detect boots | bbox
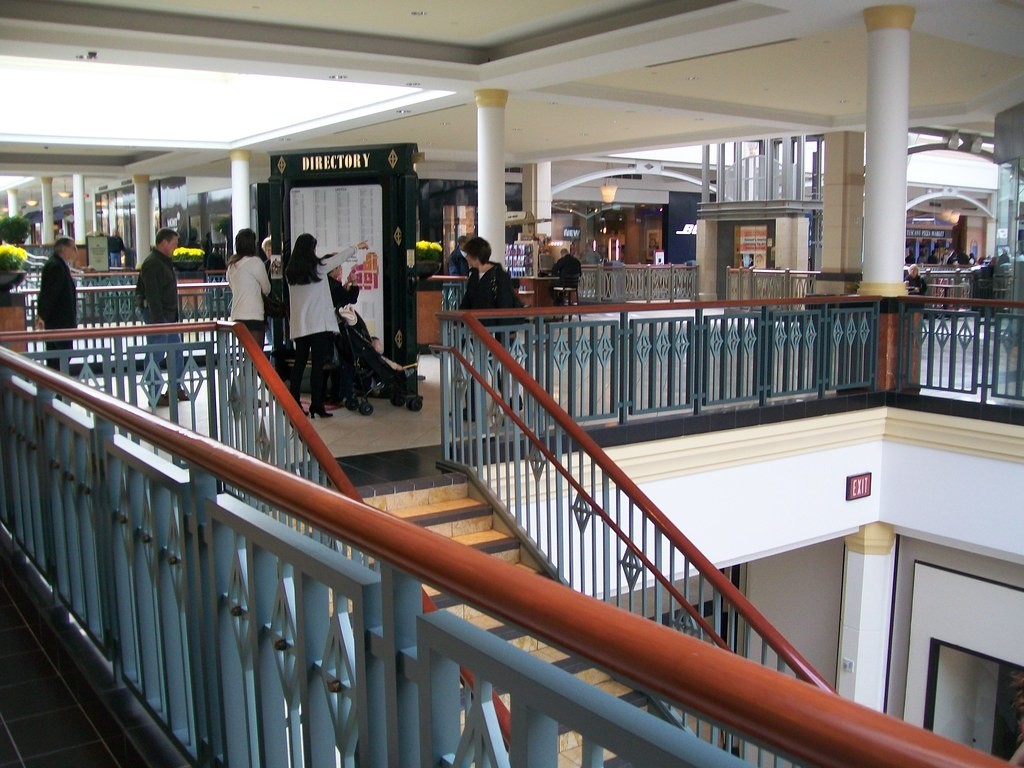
[228,376,269,408]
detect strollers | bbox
[333,305,424,416]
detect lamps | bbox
[599,161,620,204]
[949,131,960,149]
[970,134,983,154]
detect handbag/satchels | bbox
[488,266,524,334]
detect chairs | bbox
[552,273,582,322]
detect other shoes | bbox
[449,408,475,422]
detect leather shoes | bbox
[148,389,189,406]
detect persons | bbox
[108,230,125,267]
[904,264,927,309]
[550,248,582,306]
[34,236,76,403]
[225,228,405,418]
[906,249,976,265]
[447,236,531,422]
[136,226,190,407]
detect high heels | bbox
[283,402,309,416]
[309,404,333,418]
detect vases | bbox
[173,260,202,271]
[0,270,28,294]
[415,259,442,281]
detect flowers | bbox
[0,244,29,271]
[414,240,443,262]
[172,246,205,262]
